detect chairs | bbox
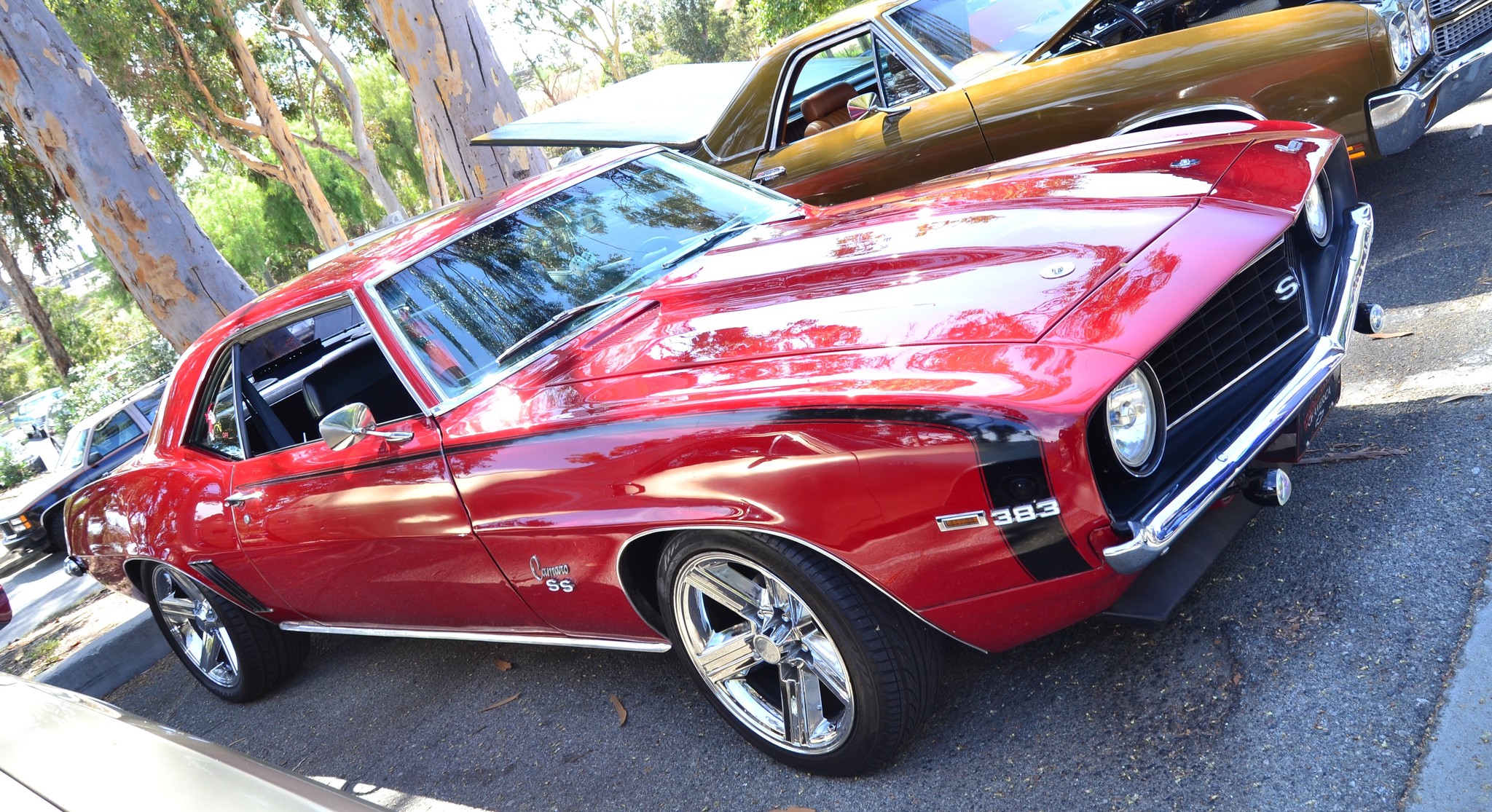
[301,325,457,435]
[801,80,859,137]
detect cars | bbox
[12,385,66,439]
[54,120,1392,782]
[460,0,1449,212]
[0,368,200,556]
